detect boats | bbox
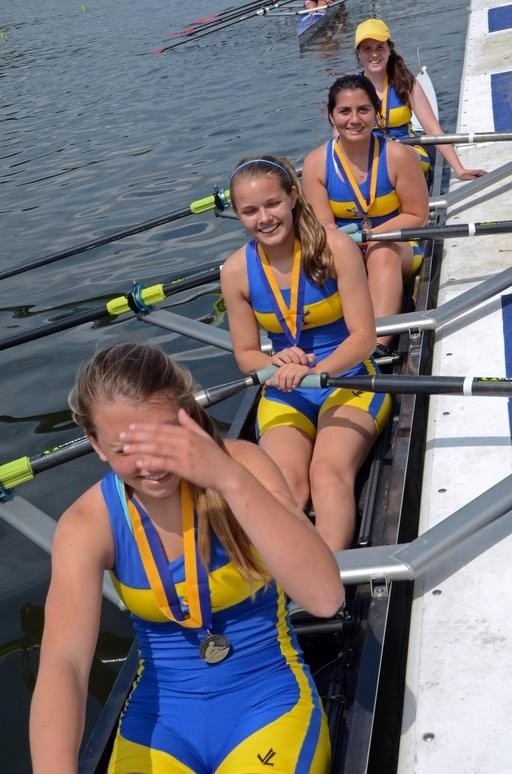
[63,62,453,774]
[294,0,342,47]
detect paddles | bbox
[153,0,292,56]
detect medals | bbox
[359,216,374,231]
[198,634,232,665]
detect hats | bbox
[353,19,392,49]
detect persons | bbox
[221,153,393,559]
[30,343,347,774]
[304,1,331,16]
[351,19,486,184]
[301,73,434,368]
